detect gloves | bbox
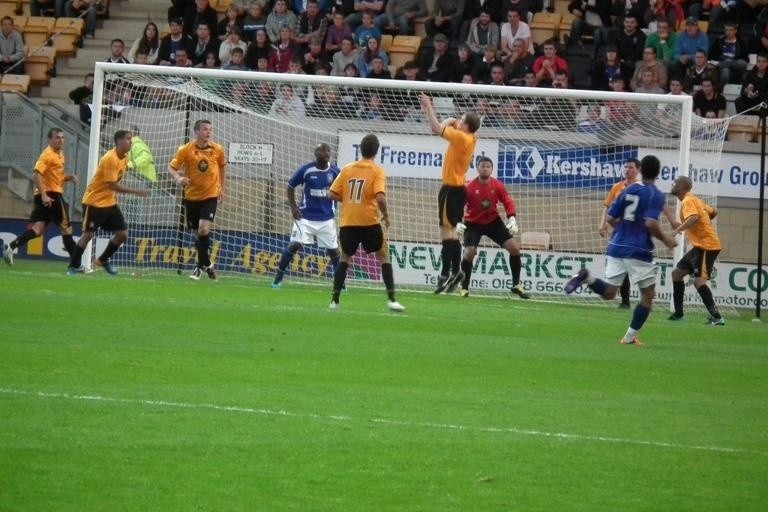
[506,216,519,236]
[456,222,467,236]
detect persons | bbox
[0,16,26,74]
[125,123,158,218]
[418,90,483,295]
[66,130,150,276]
[599,156,682,311]
[15,1,109,49]
[460,158,530,299]
[564,155,678,346]
[270,140,350,288]
[326,135,409,311]
[668,176,726,326]
[3,127,93,272]
[158,0,768,142]
[168,119,227,280]
[69,22,162,129]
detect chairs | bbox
[0,0,767,141]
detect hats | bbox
[686,16,697,24]
[434,33,448,43]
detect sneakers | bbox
[272,280,281,288]
[387,300,405,310]
[1,243,13,265]
[330,300,339,308]
[621,336,644,346]
[435,270,469,297]
[618,302,632,309]
[564,269,588,294]
[190,264,218,282]
[667,314,686,321]
[706,316,724,325]
[511,284,529,299]
[94,256,117,274]
[66,265,94,276]
